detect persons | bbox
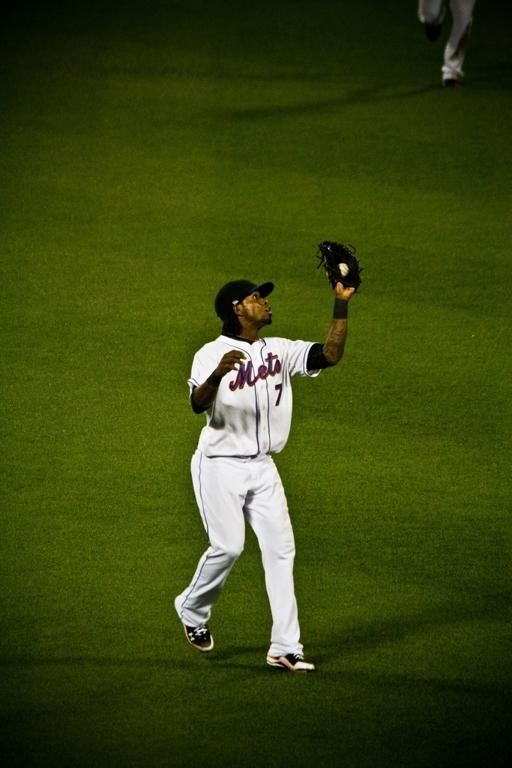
[171,240,362,671]
[416,1,475,88]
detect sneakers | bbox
[175,596,215,653]
[265,654,315,673]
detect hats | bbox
[214,278,274,320]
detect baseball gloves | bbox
[316,241,364,294]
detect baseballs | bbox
[338,263,349,277]
